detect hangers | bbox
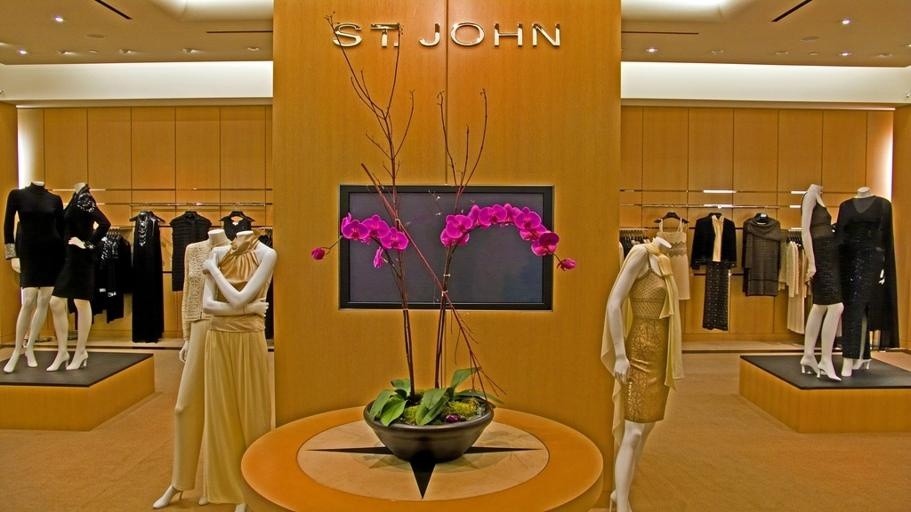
[619,226,651,244]
[784,229,806,249]
[102,226,123,244]
[653,203,776,226]
[126,206,255,224]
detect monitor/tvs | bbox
[340,184,553,309]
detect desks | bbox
[238,400,603,511]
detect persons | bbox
[201,230,277,512]
[152,228,232,510]
[800,183,845,383]
[601,234,680,512]
[3,180,67,373]
[832,185,893,377]
[46,183,112,372]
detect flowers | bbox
[305,15,581,424]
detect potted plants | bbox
[357,391,498,465]
